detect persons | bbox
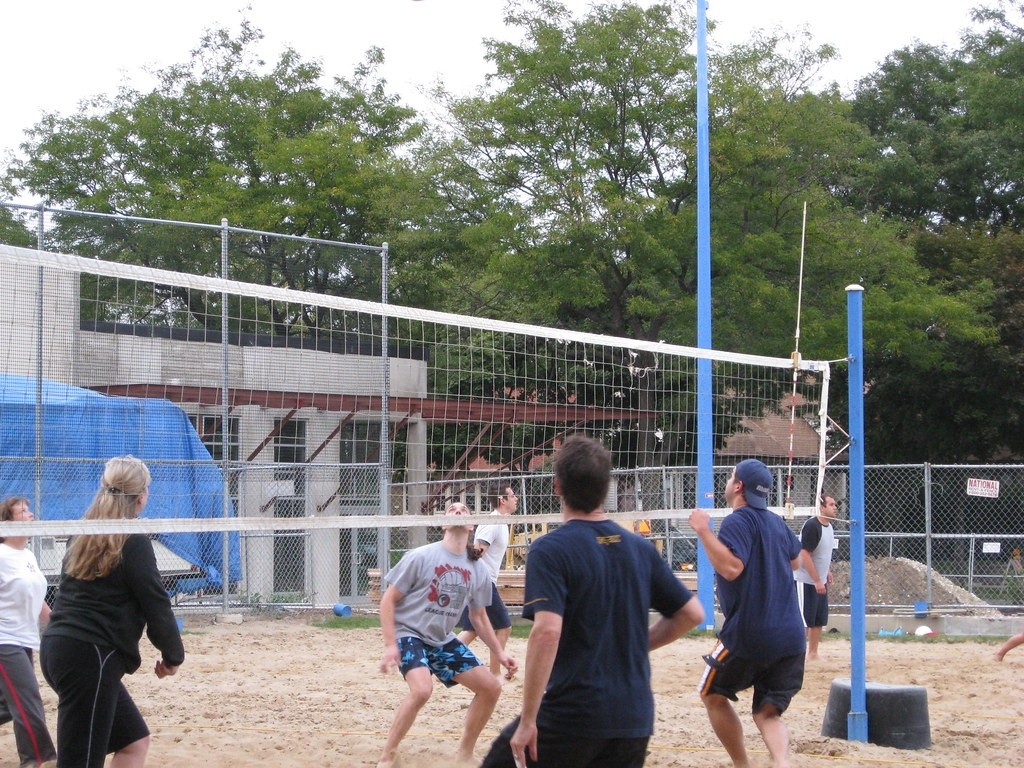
[993,630,1024,663]
[480,432,707,768]
[455,478,519,678]
[375,502,519,768]
[0,496,52,768]
[793,493,838,661]
[689,458,807,768]
[39,456,185,768]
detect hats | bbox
[736,459,771,510]
